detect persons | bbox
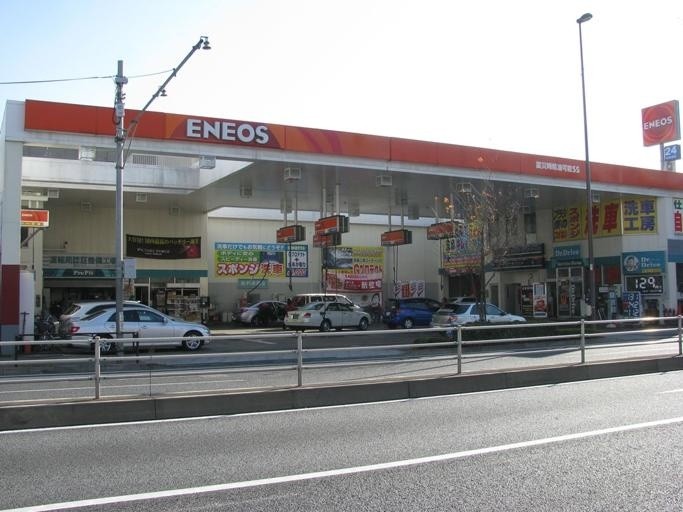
[626,256,637,271]
[363,292,382,320]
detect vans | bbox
[292,294,360,311]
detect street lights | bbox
[577,11,598,334]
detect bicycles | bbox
[34,314,55,352]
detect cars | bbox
[58,301,210,355]
[381,297,527,338]
[283,301,371,332]
[239,301,287,327]
